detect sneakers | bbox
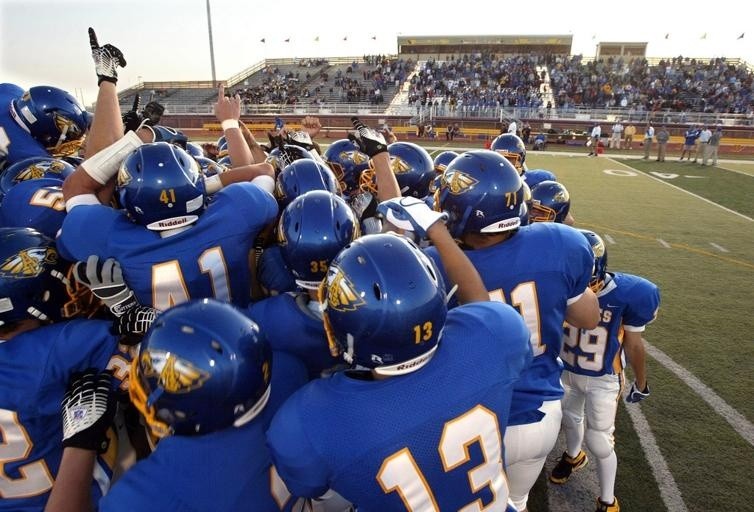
[550,448,590,484]
[594,496,620,511]
[643,157,717,167]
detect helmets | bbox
[128,297,271,436]
[0,225,68,337]
[279,190,357,288]
[322,234,449,378]
[10,86,88,147]
[575,230,605,292]
[2,133,568,235]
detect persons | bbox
[1,27,753,512]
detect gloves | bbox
[25,268,71,323]
[135,118,188,150]
[346,116,388,158]
[626,382,652,404]
[60,366,118,454]
[72,256,139,317]
[88,27,125,83]
